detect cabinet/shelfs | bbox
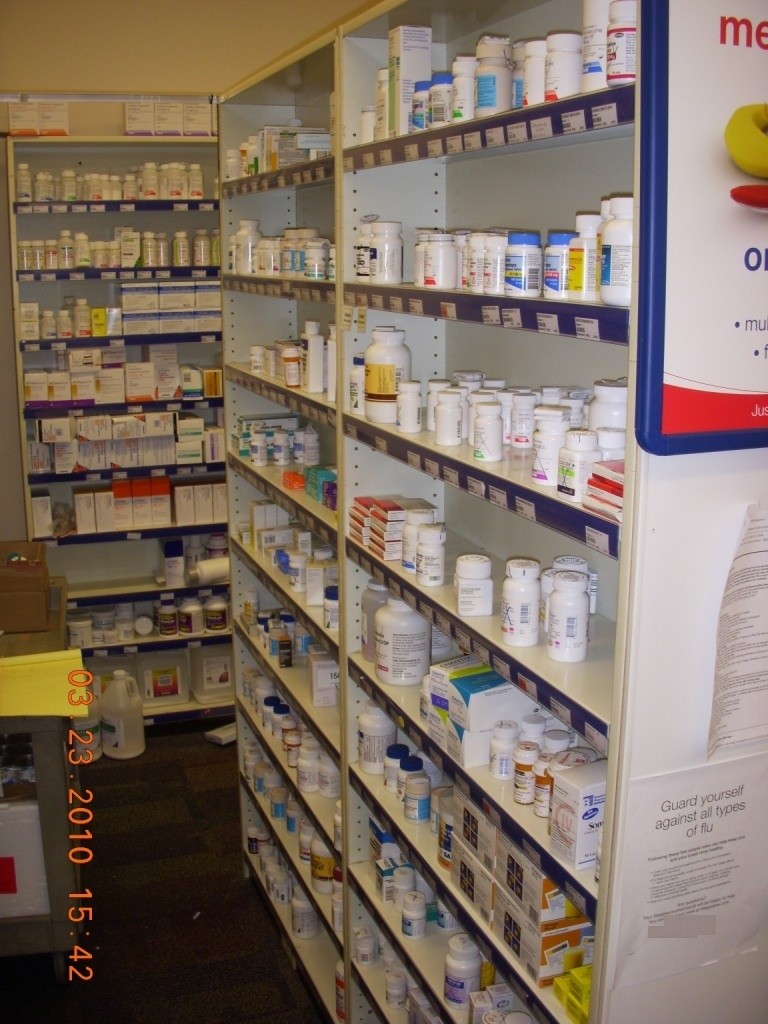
[338,0,768,1024]
[218,26,349,1024]
[5,137,239,732]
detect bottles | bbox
[287,547,339,632]
[353,196,632,308]
[410,0,638,132]
[227,219,336,282]
[67,534,230,648]
[71,668,146,762]
[40,294,91,339]
[359,508,590,686]
[248,429,321,467]
[240,668,599,1024]
[225,136,258,180]
[250,319,627,504]
[15,159,220,270]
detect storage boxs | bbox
[10,101,213,138]
[389,24,432,138]
[368,738,609,1024]
[306,643,340,709]
[1,755,79,917]
[258,122,330,171]
[0,279,226,635]
[426,653,541,766]
[82,643,237,746]
[582,460,625,521]
[232,338,337,606]
[349,495,447,562]
